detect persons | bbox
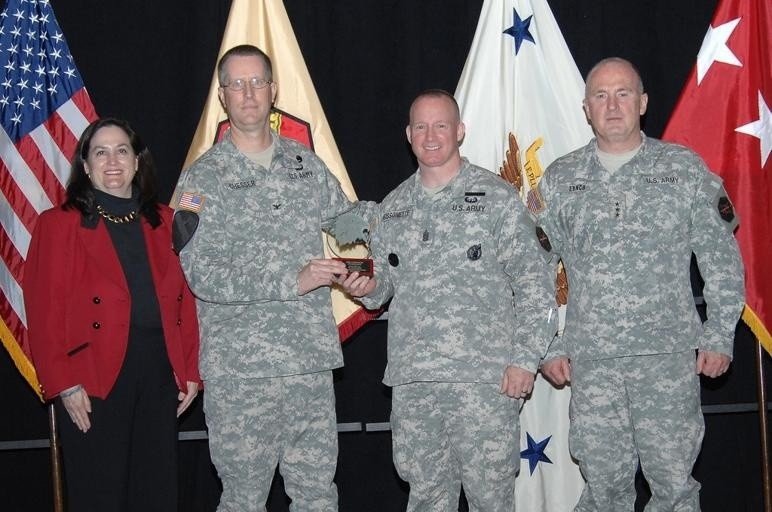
[524,58,746,512]
[334,89,559,512]
[172,43,381,512]
[23,118,199,512]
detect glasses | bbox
[223,76,271,91]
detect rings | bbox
[522,390,529,395]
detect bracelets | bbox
[60,385,86,398]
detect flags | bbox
[662,1,772,360]
[1,0,100,404]
[453,0,596,512]
[167,0,385,345]
